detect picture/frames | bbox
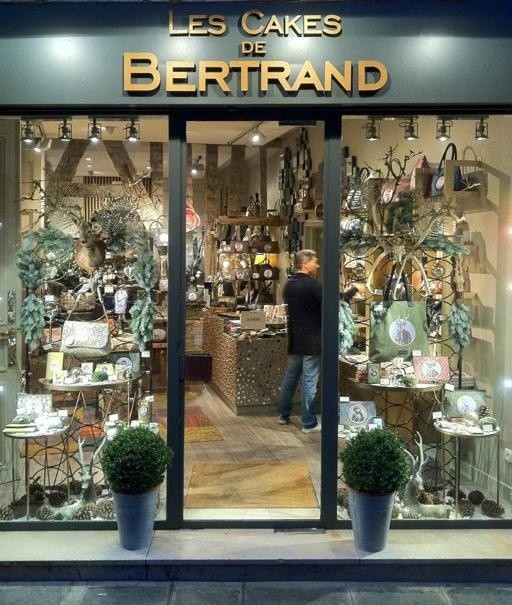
[96,363,114,375]
[367,362,382,384]
[412,356,449,382]
[80,362,93,381]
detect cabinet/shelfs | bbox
[203,216,289,318]
[339,215,412,386]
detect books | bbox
[5,421,35,428]
[1,428,37,433]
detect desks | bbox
[37,371,147,504]
[433,417,501,519]
[356,378,456,492]
[2,420,72,521]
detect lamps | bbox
[33,120,52,152]
[20,116,73,143]
[435,114,489,141]
[191,163,197,174]
[252,124,260,142]
[87,116,140,141]
[365,115,419,141]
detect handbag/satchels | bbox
[369,300,487,420]
[220,193,279,329]
[16,393,52,418]
[345,143,488,211]
[152,328,167,341]
[59,285,111,358]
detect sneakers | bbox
[279,415,289,424]
[302,424,322,433]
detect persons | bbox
[274,248,323,434]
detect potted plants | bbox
[101,423,176,550]
[339,424,414,552]
